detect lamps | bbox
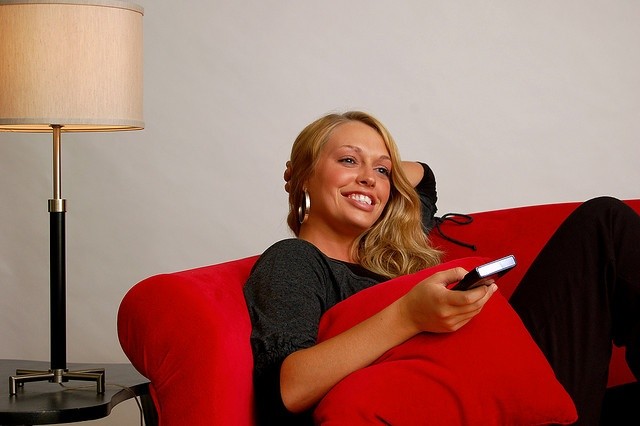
[2,1,143,411]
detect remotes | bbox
[450,255,516,290]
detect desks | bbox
[1,359,158,426]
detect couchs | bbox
[117,200,640,425]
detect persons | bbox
[243,110,640,423]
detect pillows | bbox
[311,256,579,424]
